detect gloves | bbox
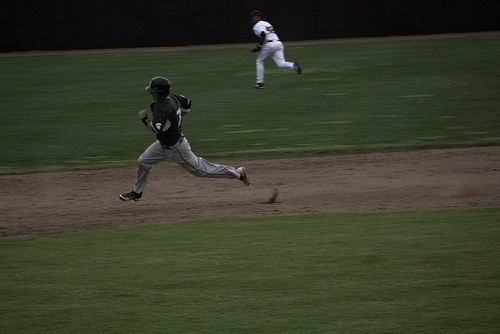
[250,45,262,53]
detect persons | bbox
[120,76,251,202]
[249,11,302,88]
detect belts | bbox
[266,40,281,42]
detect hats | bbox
[248,10,262,18]
[145,77,172,93]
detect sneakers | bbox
[237,166,250,186]
[253,84,265,89]
[294,63,301,74]
[119,190,143,201]
